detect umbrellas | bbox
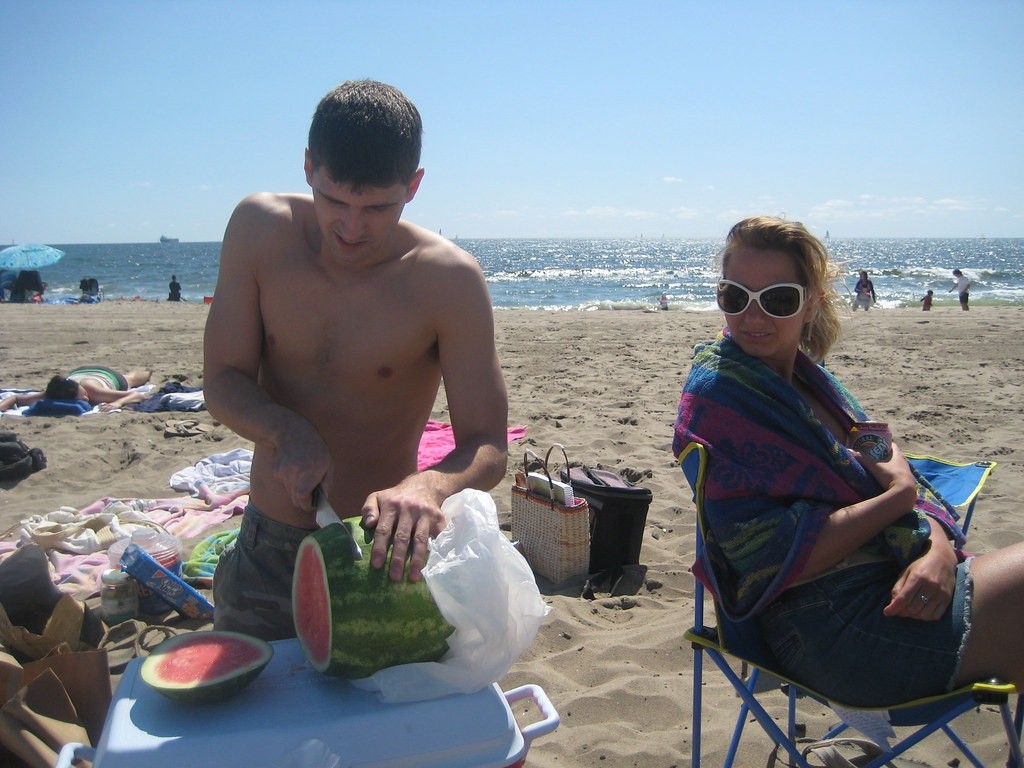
[0,242,65,304]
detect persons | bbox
[203,78,508,642]
[851,271,876,312]
[79,278,102,303]
[167,275,181,302]
[657,292,668,310]
[949,269,971,311]
[920,290,933,311]
[0,365,153,412]
[672,223,1024,726]
[0,262,47,302]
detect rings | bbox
[917,592,928,605]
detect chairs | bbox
[678,441,1024,768]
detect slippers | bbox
[776,739,898,768]
[97,619,147,672]
[166,420,215,436]
[135,625,193,658]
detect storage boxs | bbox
[55,637,560,768]
[560,468,653,573]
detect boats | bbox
[160,235,180,244]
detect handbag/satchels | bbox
[0,543,110,665]
[347,488,553,703]
[561,465,653,575]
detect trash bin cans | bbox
[559,466,654,577]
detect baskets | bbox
[511,444,591,584]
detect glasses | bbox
[717,280,806,318]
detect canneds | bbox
[849,420,892,462]
[100,568,139,624]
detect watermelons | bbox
[292,514,456,679]
[140,630,275,705]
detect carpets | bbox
[178,528,241,588]
